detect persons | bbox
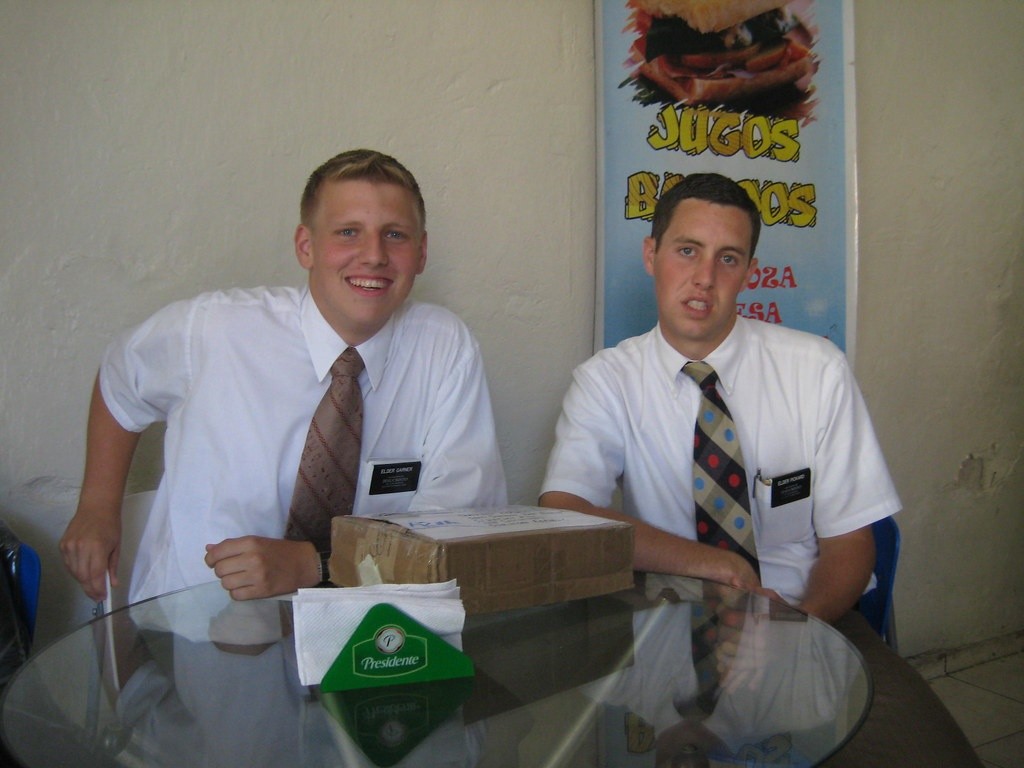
[537,173,982,768]
[58,146,508,768]
[96,596,865,768]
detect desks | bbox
[0,574,877,768]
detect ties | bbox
[284,346,365,559]
[677,600,746,721]
[682,361,761,585]
[278,599,302,711]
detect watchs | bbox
[310,537,332,584]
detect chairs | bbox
[850,516,901,657]
[80,489,158,749]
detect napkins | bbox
[327,713,484,768]
[293,578,466,686]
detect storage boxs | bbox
[328,506,634,616]
[457,595,635,725]
